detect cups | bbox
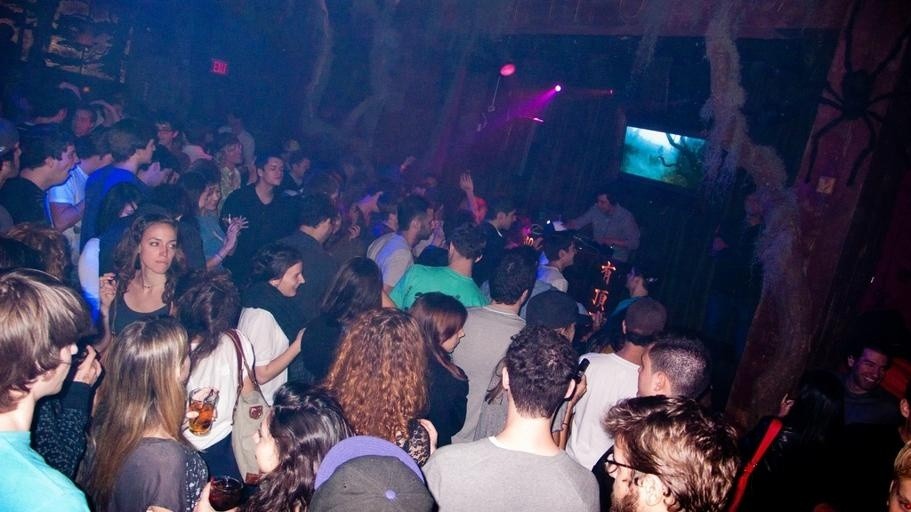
[188,386,217,435]
[209,475,244,508]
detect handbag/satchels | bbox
[231,386,285,485]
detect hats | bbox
[307,434,436,512]
[524,289,593,330]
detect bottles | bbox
[565,357,591,400]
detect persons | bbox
[1,20,910,511]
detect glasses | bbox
[603,452,648,475]
